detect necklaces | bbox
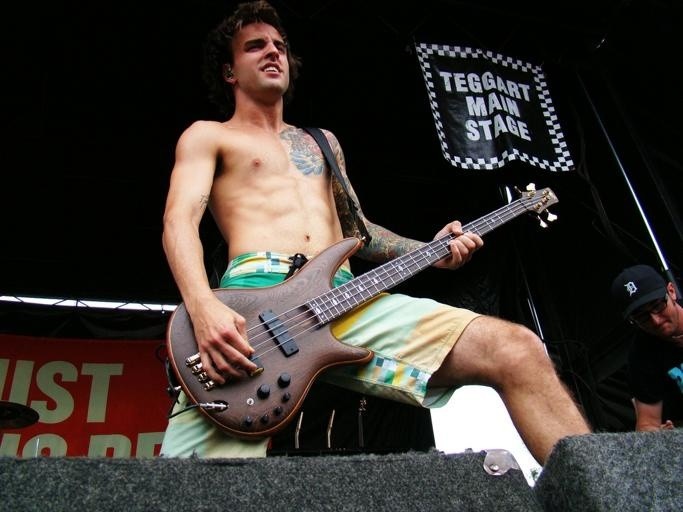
[669,333,682,339]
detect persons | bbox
[160,1,593,466]
[609,264,682,433]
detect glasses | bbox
[624,293,673,324]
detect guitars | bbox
[167,184,560,440]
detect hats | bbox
[612,263,669,316]
